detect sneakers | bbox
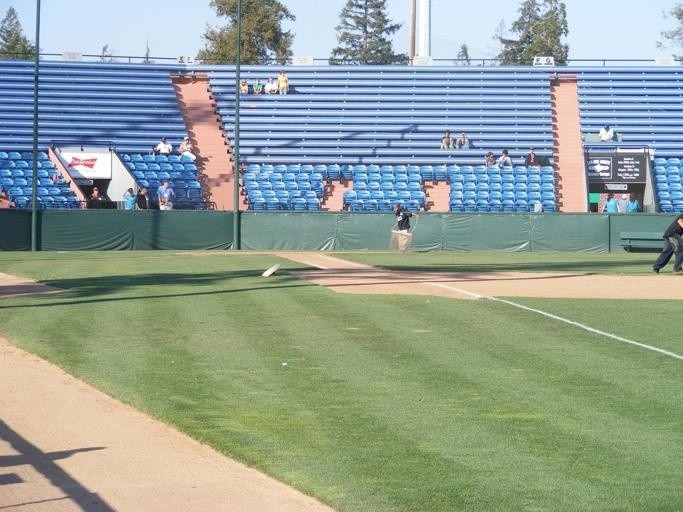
[652,264,659,274]
[672,270,683,275]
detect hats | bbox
[392,203,400,211]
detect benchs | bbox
[0,60,193,153]
[576,66,683,158]
[193,65,555,163]
[619,232,665,251]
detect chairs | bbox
[240,164,558,212]
[650,158,683,213]
[1,153,81,212]
[115,154,204,211]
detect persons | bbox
[122,187,137,211]
[276,69,289,96]
[86,187,106,201]
[153,137,172,155]
[239,80,248,95]
[651,214,682,275]
[135,188,148,210]
[601,191,621,213]
[392,203,419,251]
[440,130,453,149]
[179,136,197,161]
[263,77,277,94]
[251,79,262,95]
[484,151,496,167]
[496,149,513,167]
[156,180,176,211]
[454,133,470,150]
[523,145,540,168]
[624,191,640,213]
[598,125,614,142]
